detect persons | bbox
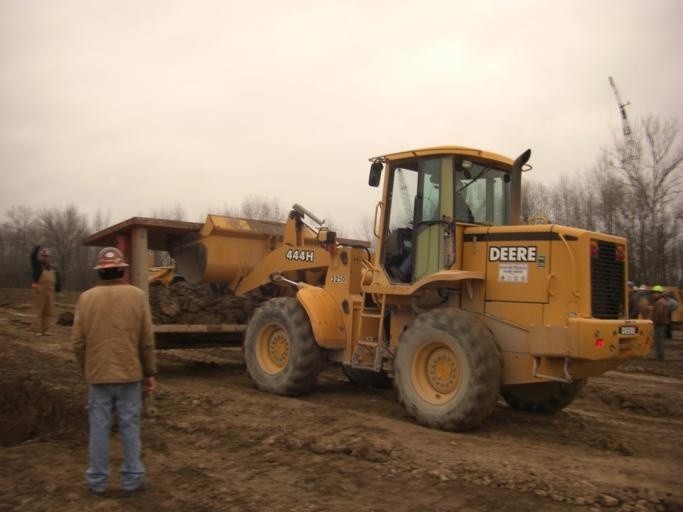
[72,247,155,493]
[29,241,63,330]
[390,177,466,283]
[627,279,678,360]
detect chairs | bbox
[456,210,475,223]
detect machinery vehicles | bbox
[627,283,683,327]
[80,146,658,430]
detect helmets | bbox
[626,281,663,294]
[40,248,50,257]
[92,246,129,271]
